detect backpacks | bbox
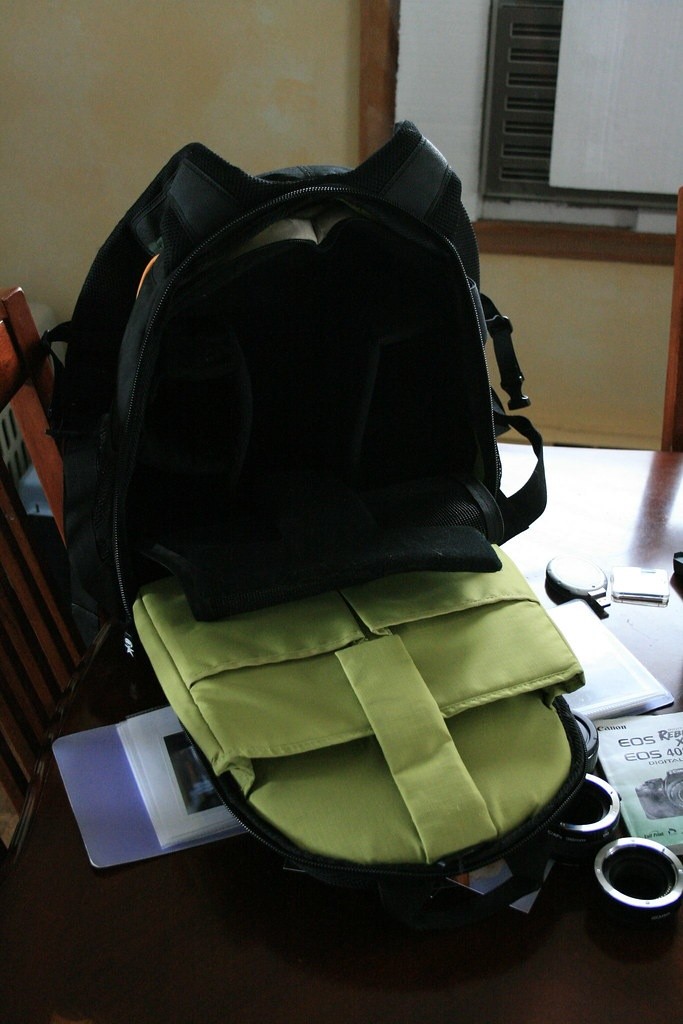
[0,117,551,622]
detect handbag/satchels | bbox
[126,540,605,923]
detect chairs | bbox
[1,284,114,874]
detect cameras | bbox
[636,768,683,820]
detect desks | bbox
[1,443,683,1024]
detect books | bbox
[446,854,555,913]
[49,704,248,870]
[591,712,683,858]
[541,598,676,720]
